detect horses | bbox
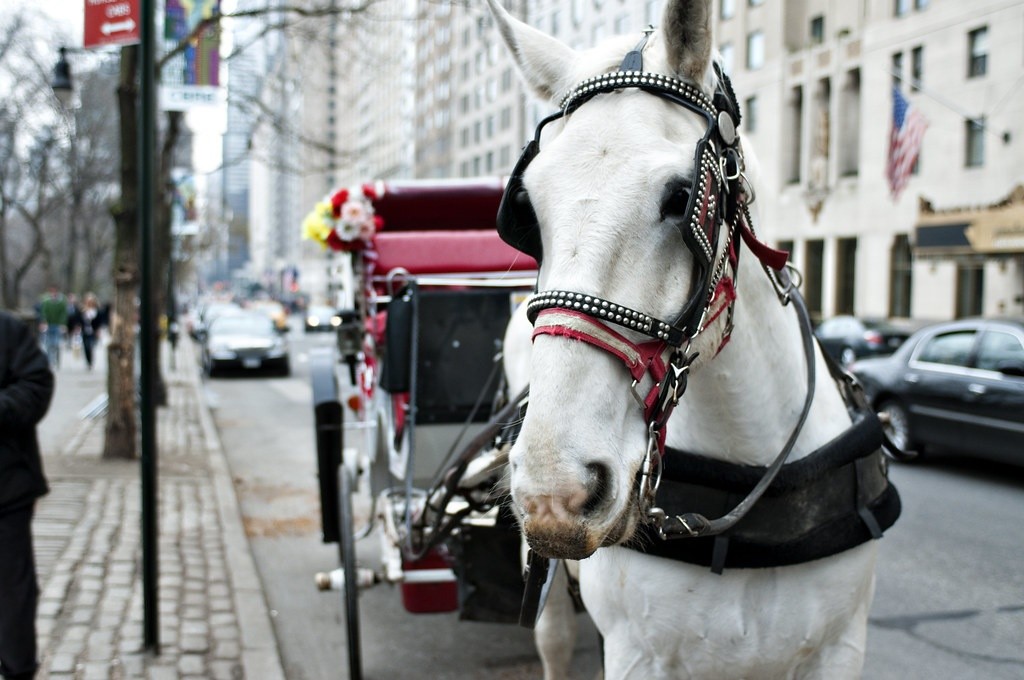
[487,0,882,679]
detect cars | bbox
[304,305,342,332]
[186,300,291,379]
[813,314,1024,468]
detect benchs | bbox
[368,230,539,423]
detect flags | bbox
[887,86,928,200]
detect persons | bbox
[39,283,110,367]
[0,308,55,680]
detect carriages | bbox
[302,1,903,679]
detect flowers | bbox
[301,177,384,256]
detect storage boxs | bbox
[400,548,459,615]
